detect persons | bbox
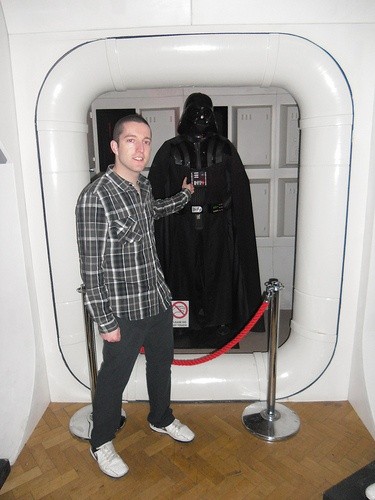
[75,115,194,478]
[148,92,266,349]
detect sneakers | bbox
[90,440,129,478]
[149,418,195,443]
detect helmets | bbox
[177,92,220,134]
